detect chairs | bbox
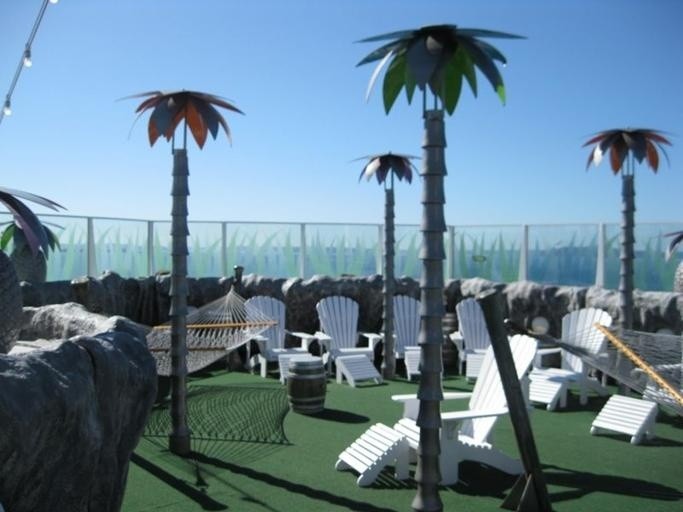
[590,364,683,445]
[379,294,423,382]
[335,334,539,487]
[529,307,612,413]
[314,295,383,387]
[449,297,493,381]
[243,295,314,378]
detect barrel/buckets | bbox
[287,355,326,413]
